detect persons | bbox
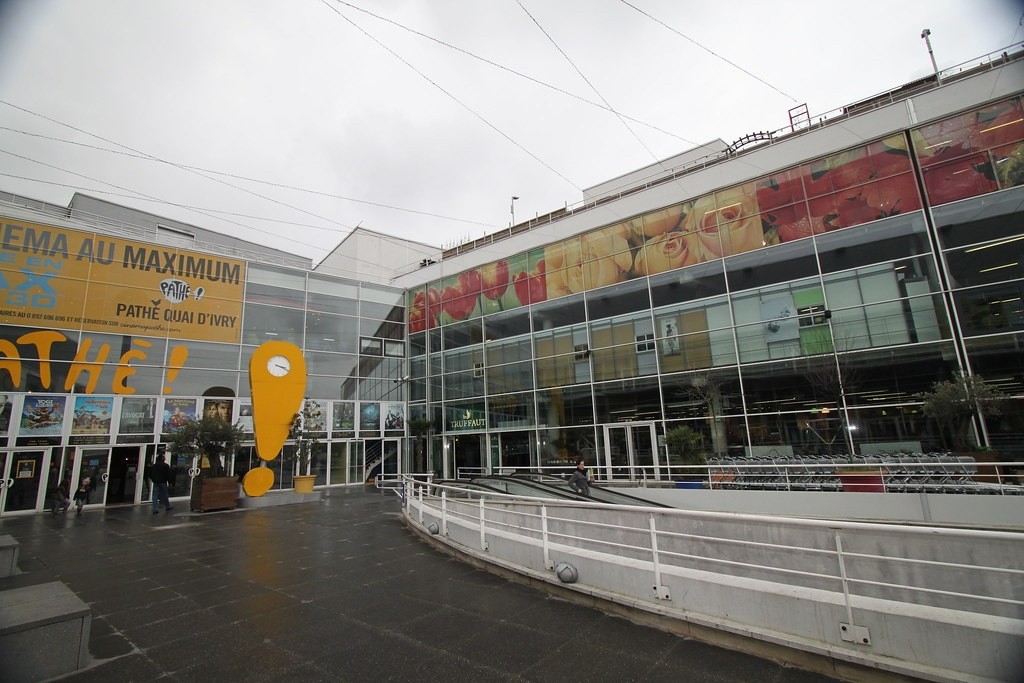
[48,477,91,519]
[148,455,177,515]
[386,410,402,429]
[568,459,591,501]
[45,460,58,492]
[665,324,677,353]
[137,395,233,431]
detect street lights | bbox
[922,27,942,86]
[511,193,520,227]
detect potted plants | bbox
[664,425,711,489]
[289,397,322,492]
[168,415,246,514]
[910,370,1009,483]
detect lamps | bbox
[824,310,831,318]
[586,350,591,355]
